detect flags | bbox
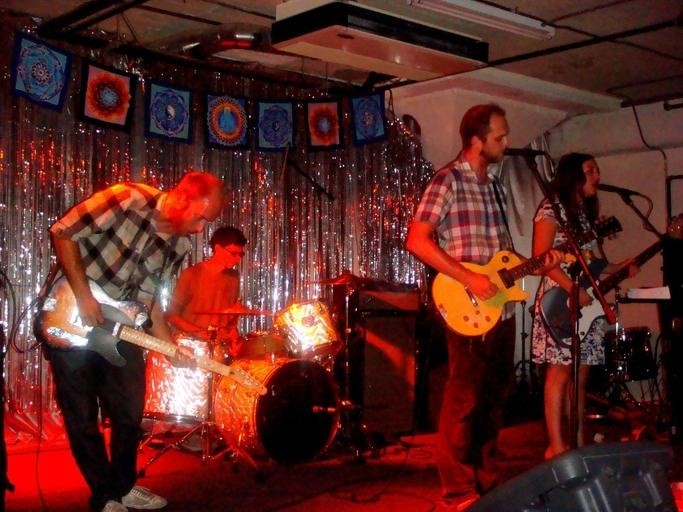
[76,64,137,133]
[146,81,196,143]
[257,101,291,151]
[350,93,387,143]
[308,102,343,148]
[16,38,72,112]
[201,94,252,152]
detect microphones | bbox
[596,182,638,196]
[504,146,547,158]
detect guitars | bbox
[539,220,683,349]
[431,215,624,337]
[33,275,268,397]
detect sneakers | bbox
[120,486,168,509]
[101,500,128,512]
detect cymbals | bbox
[193,298,271,315]
[305,269,388,286]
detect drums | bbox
[602,326,657,383]
[211,353,341,470]
[243,330,288,360]
[144,333,213,424]
[273,300,344,367]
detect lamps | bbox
[407,0,555,42]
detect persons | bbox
[35,170,222,512]
[165,226,246,354]
[531,153,641,467]
[405,103,565,512]
